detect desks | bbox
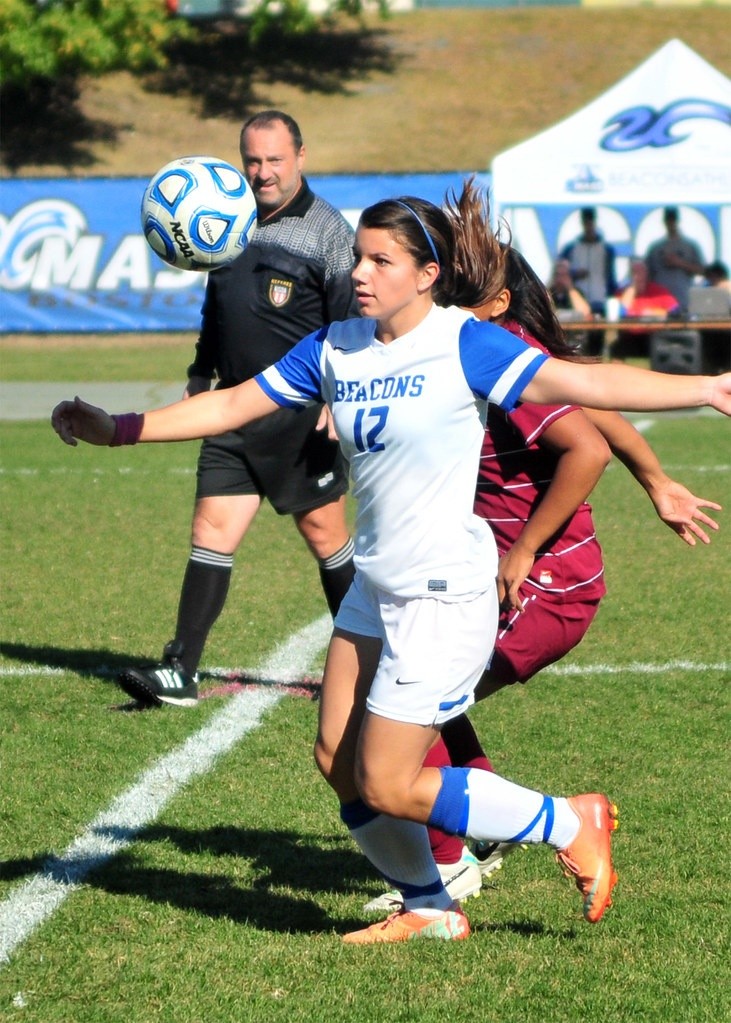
[557,312,731,363]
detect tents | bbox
[493,36,731,242]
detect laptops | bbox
[686,287,731,322]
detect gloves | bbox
[51,396,144,447]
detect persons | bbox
[645,206,708,307]
[116,110,361,707]
[608,259,680,362]
[557,207,619,357]
[544,261,590,355]
[669,261,731,376]
[364,242,723,912]
[52,196,731,944]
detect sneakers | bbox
[469,840,503,878]
[116,639,198,707]
[363,844,482,912]
[555,793,618,924]
[342,899,469,943]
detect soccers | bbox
[139,152,260,273]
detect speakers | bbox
[651,330,703,376]
